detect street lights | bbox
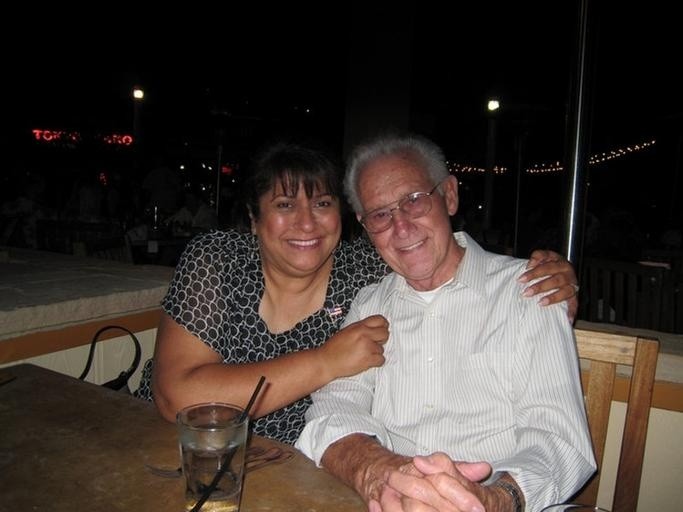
[129,84,166,237]
[483,96,525,251]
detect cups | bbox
[174,401,249,512]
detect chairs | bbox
[571,328,661,512]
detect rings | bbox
[569,283,581,294]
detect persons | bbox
[290,133,599,511]
[131,137,393,448]
[165,180,219,235]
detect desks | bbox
[0,362,378,511]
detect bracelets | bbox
[491,480,520,512]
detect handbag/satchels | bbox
[101,378,132,394]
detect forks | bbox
[144,446,264,479]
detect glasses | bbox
[359,175,448,235]
